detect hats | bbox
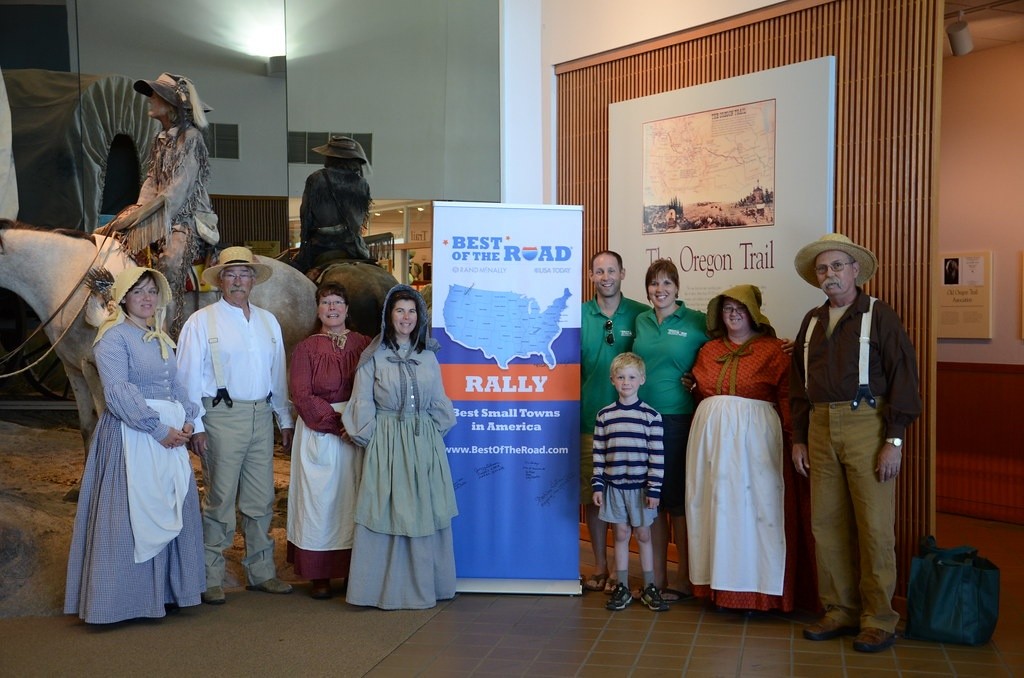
[312,136,374,178]
[91,266,177,361]
[705,284,777,395]
[202,246,272,286]
[356,283,441,437]
[133,71,214,130]
[794,233,877,288]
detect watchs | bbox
[885,438,903,446]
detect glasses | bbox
[221,271,253,282]
[318,300,346,306]
[605,320,614,348]
[721,304,748,314]
[813,260,857,276]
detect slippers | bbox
[658,588,694,603]
[586,572,610,592]
[603,579,619,594]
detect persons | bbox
[289,136,376,273]
[591,353,669,610]
[632,259,795,603]
[343,284,459,611]
[285,282,373,598]
[685,285,804,617]
[581,251,696,594]
[94,73,213,337]
[65,267,207,624]
[176,247,294,604]
[788,234,920,650]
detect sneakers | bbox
[606,583,631,609]
[641,583,669,611]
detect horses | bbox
[1,219,318,501]
[279,247,399,339]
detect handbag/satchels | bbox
[904,535,999,647]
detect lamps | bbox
[947,10,974,56]
[268,55,286,78]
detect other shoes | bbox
[245,578,292,593]
[802,616,859,640]
[203,586,225,603]
[311,579,332,598]
[853,631,896,653]
